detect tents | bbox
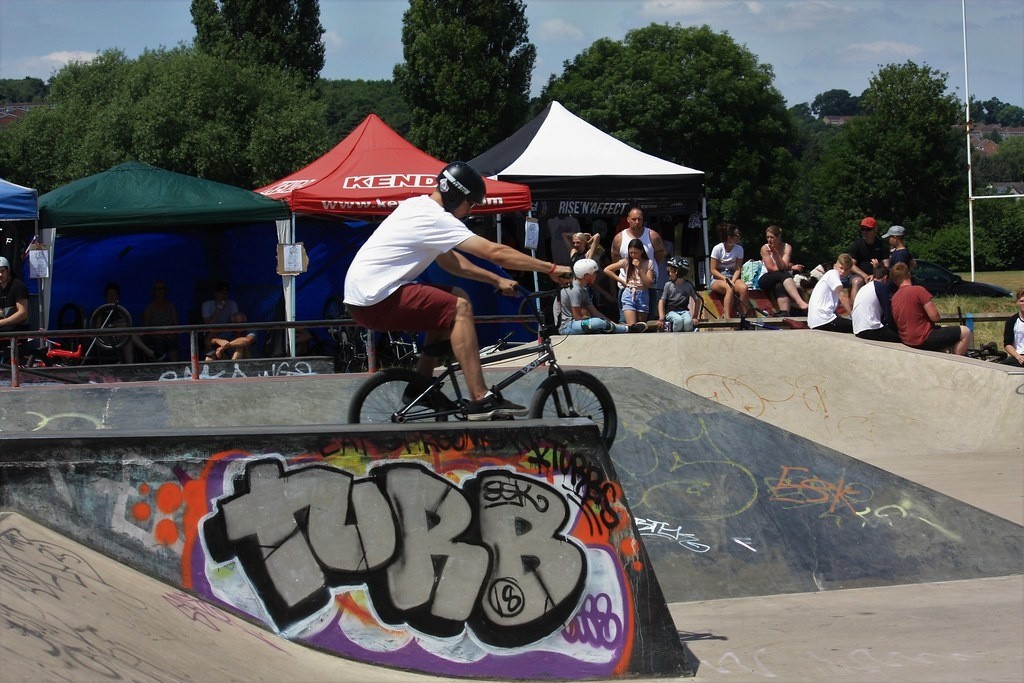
[38,162,293,356]
[463,100,710,290]
[252,115,543,355]
[0,178,46,346]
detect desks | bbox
[697,288,854,332]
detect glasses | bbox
[730,233,739,237]
[155,287,166,290]
[467,199,475,208]
[860,227,874,232]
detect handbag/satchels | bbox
[740,259,763,290]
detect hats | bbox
[882,225,905,238]
[860,216,877,228]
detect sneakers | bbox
[625,321,647,333]
[466,384,529,421]
[403,378,458,412]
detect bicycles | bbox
[955,307,1008,363]
[692,278,807,331]
[0,300,134,373]
[327,326,422,372]
[348,272,619,452]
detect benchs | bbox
[647,312,1015,350]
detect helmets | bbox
[573,258,598,279]
[666,256,689,278]
[435,162,486,214]
[0,257,11,272]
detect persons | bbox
[0,256,30,351]
[553,208,700,335]
[343,163,574,421]
[96,285,166,363]
[267,311,311,355]
[143,283,180,362]
[711,226,749,319]
[1001,289,1024,367]
[758,226,809,314]
[807,217,970,354]
[201,282,257,360]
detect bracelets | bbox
[549,264,556,273]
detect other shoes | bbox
[773,310,789,317]
[149,351,166,361]
